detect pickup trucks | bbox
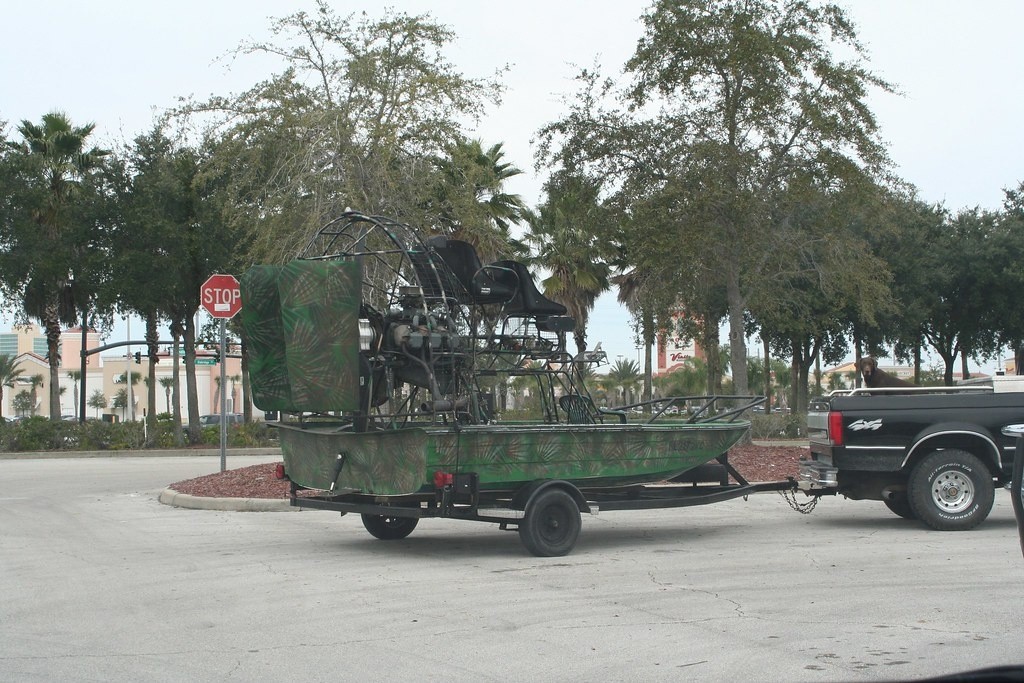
[798,342,1024,531]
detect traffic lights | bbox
[136,352,140,363]
[183,356,186,363]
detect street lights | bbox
[635,341,644,403]
[617,355,624,364]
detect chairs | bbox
[410,238,520,304]
[490,260,567,317]
[559,394,628,424]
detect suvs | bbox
[182,414,243,439]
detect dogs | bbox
[853,358,931,395]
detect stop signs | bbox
[201,274,242,318]
